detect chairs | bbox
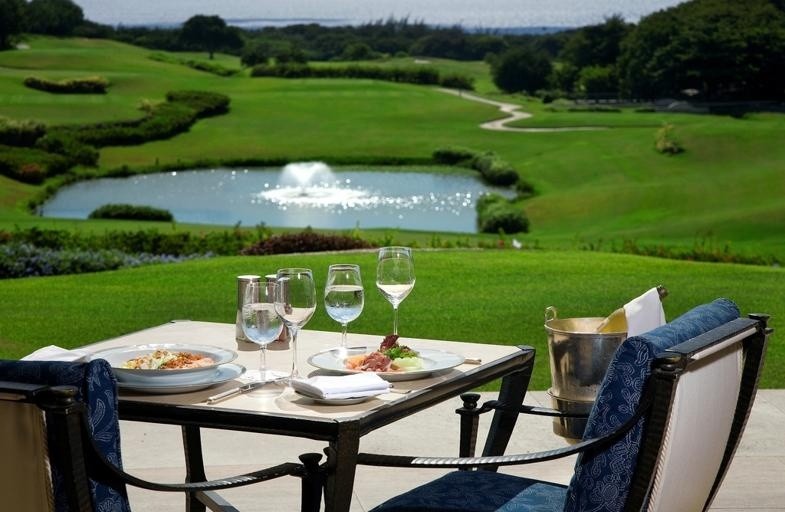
[355,298,774,510]
[0,354,324,511]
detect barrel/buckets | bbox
[540,307,628,437]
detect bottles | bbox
[597,286,667,333]
[235,275,262,342]
[265,274,290,340]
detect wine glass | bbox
[275,268,317,387]
[243,281,281,395]
[324,265,363,349]
[376,246,417,347]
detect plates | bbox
[85,342,240,382]
[292,389,382,403]
[307,342,465,380]
[112,363,246,394]
